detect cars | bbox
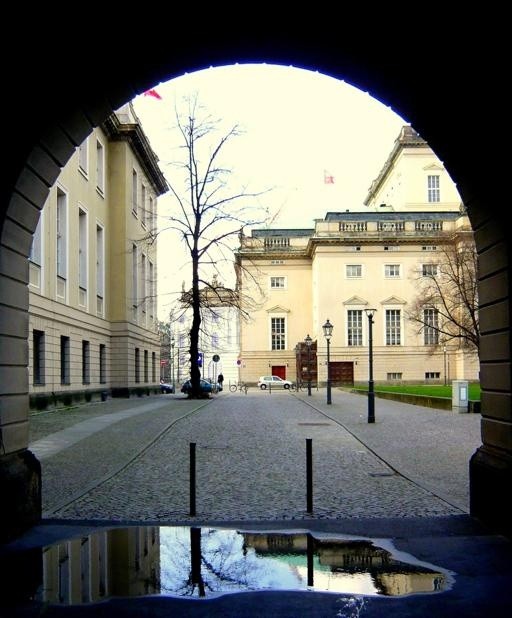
[160,377,222,394]
[257,376,294,390]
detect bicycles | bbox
[289,380,308,393]
[229,382,249,393]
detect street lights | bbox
[363,307,378,425]
[443,343,448,386]
[321,319,335,405]
[304,334,314,397]
